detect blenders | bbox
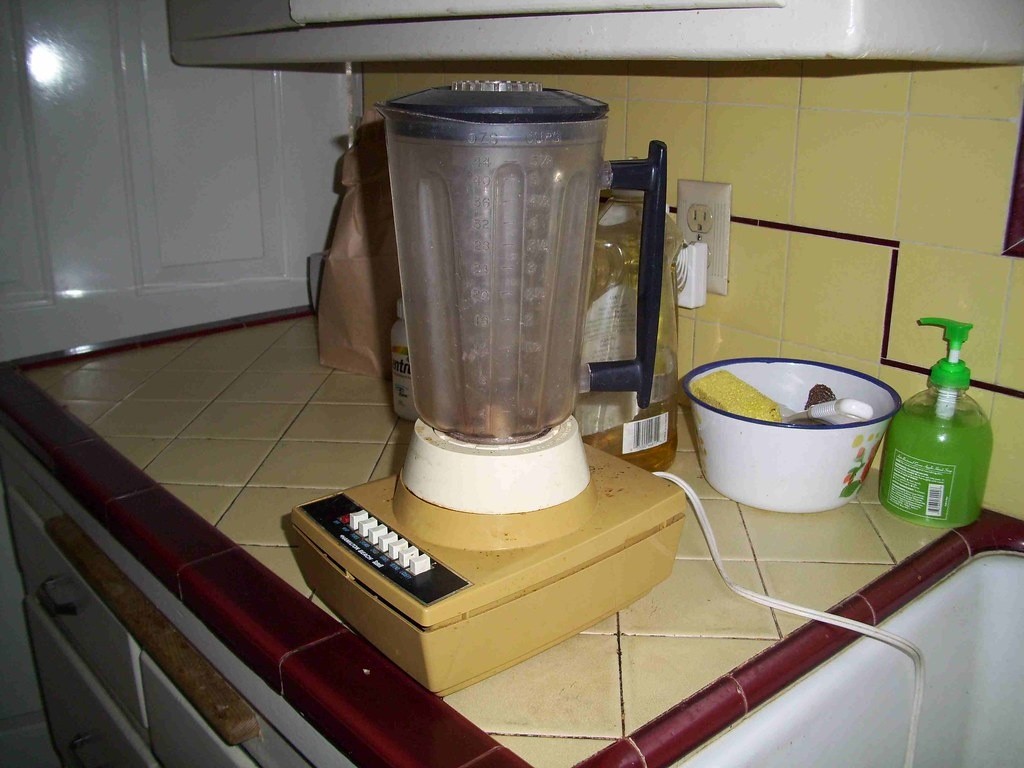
[284,79,683,696]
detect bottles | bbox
[574,157,681,470]
[391,297,417,421]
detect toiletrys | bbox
[878,315,994,531]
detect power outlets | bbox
[674,178,733,293]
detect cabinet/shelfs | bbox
[0,414,981,768]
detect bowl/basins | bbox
[683,357,900,512]
